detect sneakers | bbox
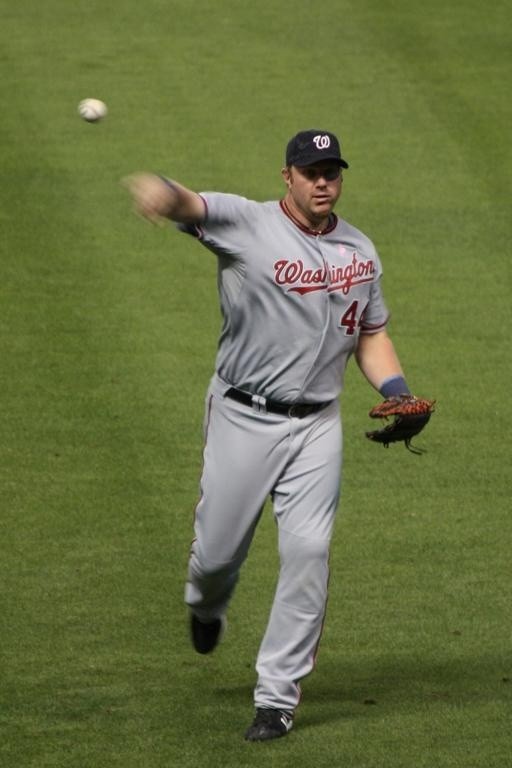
[191,611,223,654]
[246,706,294,740]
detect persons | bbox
[123,129,430,744]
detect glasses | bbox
[298,163,337,181]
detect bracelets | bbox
[377,373,408,398]
[156,168,182,215]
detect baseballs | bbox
[78,98,107,122]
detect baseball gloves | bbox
[366,393,437,454]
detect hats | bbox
[286,130,350,169]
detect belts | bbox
[227,387,332,416]
